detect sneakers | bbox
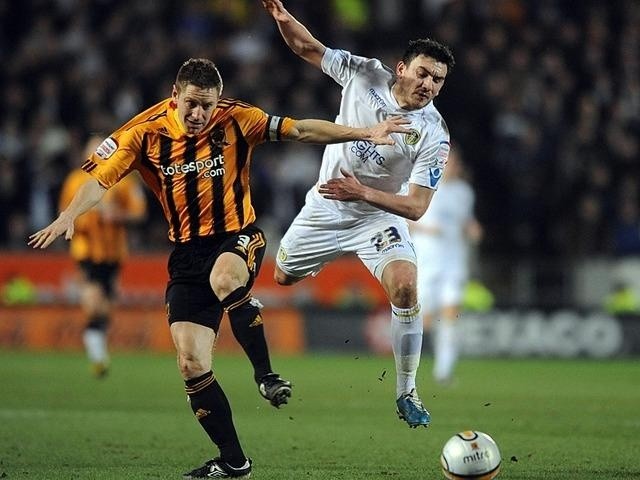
[259,372,292,408]
[186,457,252,479]
[395,388,431,429]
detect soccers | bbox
[441,431,501,480]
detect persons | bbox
[58,129,150,376]
[25,58,411,478]
[1,0,640,257]
[396,137,484,384]
[260,0,455,429]
